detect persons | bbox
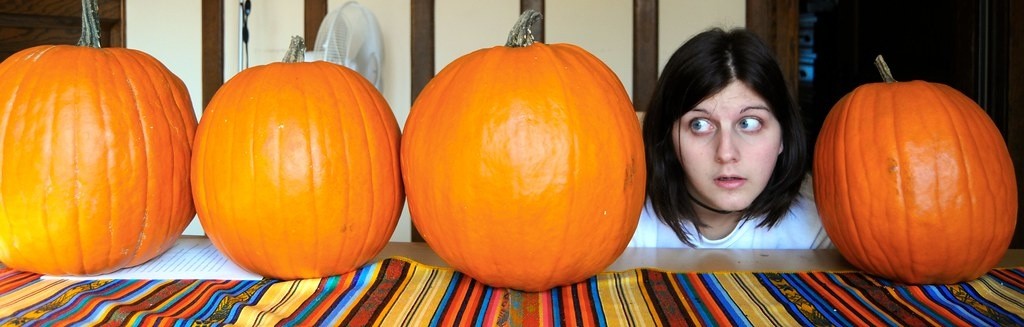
[627,27,835,249]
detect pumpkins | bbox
[812,53,1019,283]
[0,7,646,290]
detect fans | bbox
[299,0,385,94]
